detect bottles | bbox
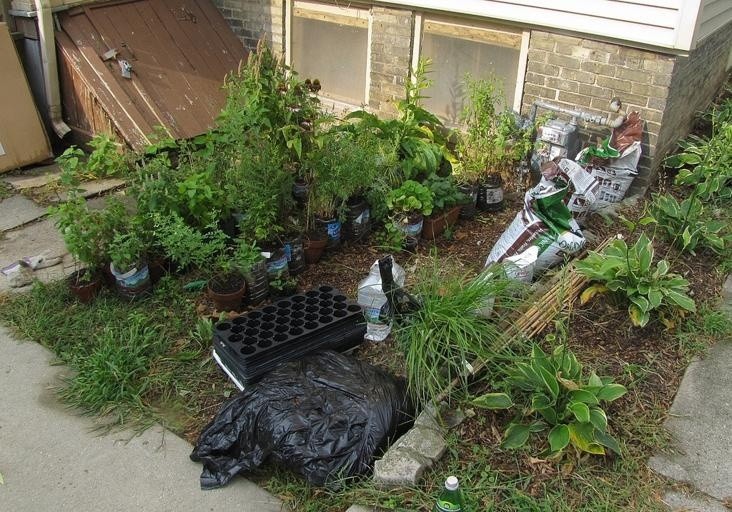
[431,475,465,511]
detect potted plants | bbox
[51,31,556,312]
